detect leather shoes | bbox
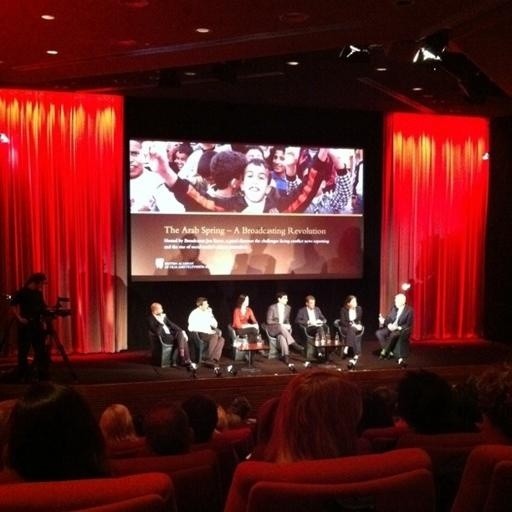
[379,351,395,361]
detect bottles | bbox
[315,332,331,347]
[334,331,340,346]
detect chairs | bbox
[147,325,412,369]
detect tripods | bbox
[37,320,77,382]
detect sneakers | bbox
[256,349,269,357]
[281,355,290,364]
[317,354,326,361]
[353,355,359,360]
[178,360,197,370]
[343,348,348,354]
[292,345,305,352]
[205,359,220,367]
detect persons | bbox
[148,302,193,371]
[232,293,268,363]
[8,273,50,365]
[266,290,304,363]
[340,294,364,361]
[130,139,364,217]
[294,294,330,361]
[374,293,414,365]
[187,296,226,367]
[2,366,512,512]
